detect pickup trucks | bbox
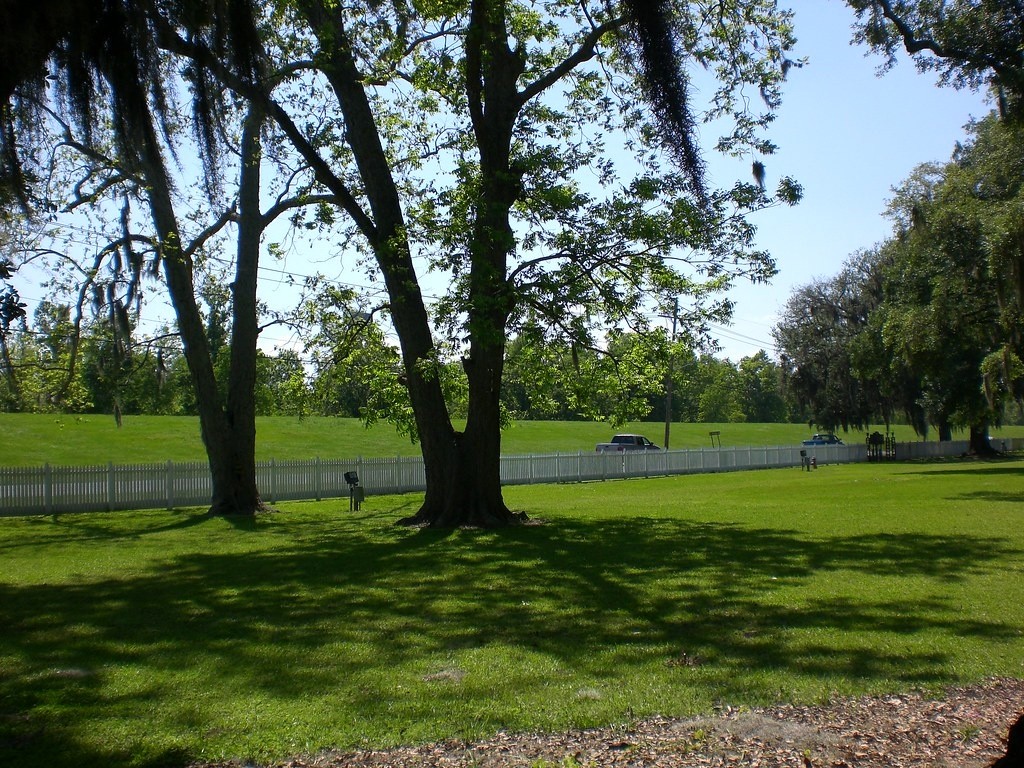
[596,434,661,452]
[802,434,844,445]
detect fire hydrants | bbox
[811,456,817,469]
